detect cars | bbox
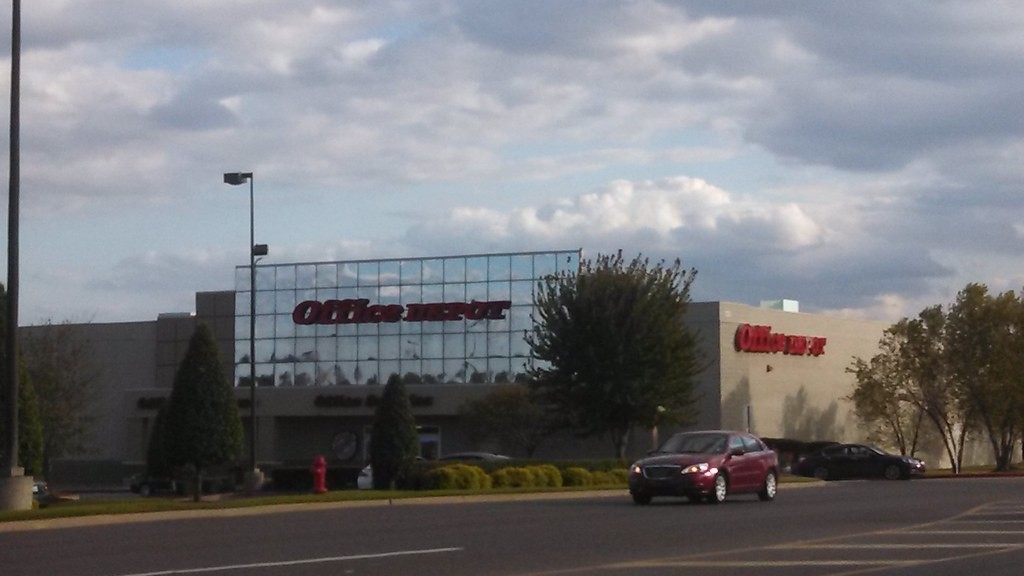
[126,463,228,499]
[357,454,432,491]
[790,442,928,481]
[627,429,782,505]
[23,470,50,507]
[436,450,512,474]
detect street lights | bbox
[221,171,271,470]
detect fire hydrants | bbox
[311,455,329,496]
